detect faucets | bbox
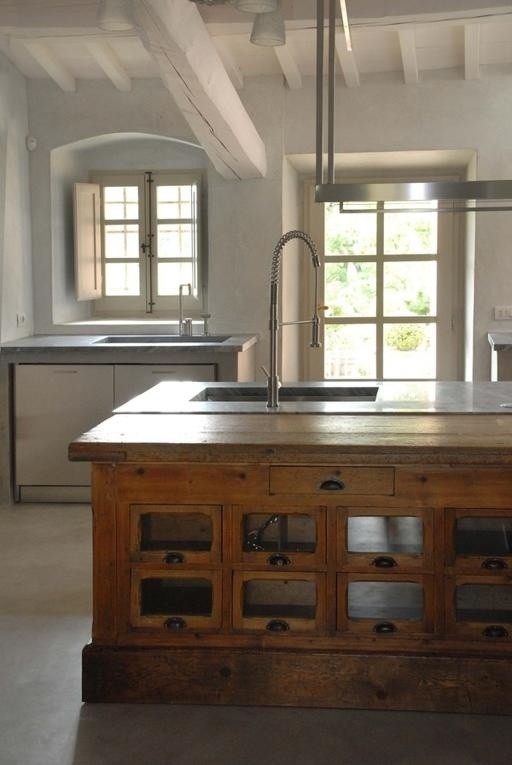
[177,283,192,335]
[258,229,322,408]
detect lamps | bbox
[97,1,135,33]
[234,1,286,48]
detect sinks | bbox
[93,335,232,343]
[189,386,380,403]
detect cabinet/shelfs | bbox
[67,412,511,717]
